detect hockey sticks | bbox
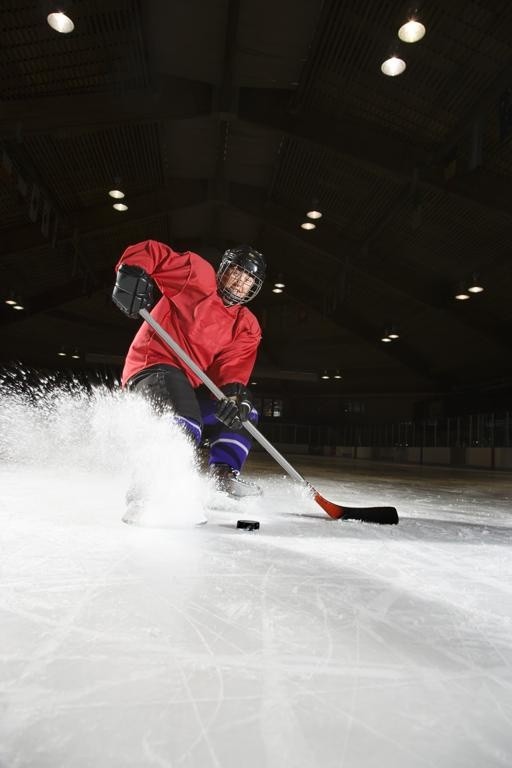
[138,304,398,526]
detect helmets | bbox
[215,245,269,305]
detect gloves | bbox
[111,269,155,322]
[213,382,254,432]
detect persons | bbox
[113,232,264,537]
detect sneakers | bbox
[207,464,264,502]
